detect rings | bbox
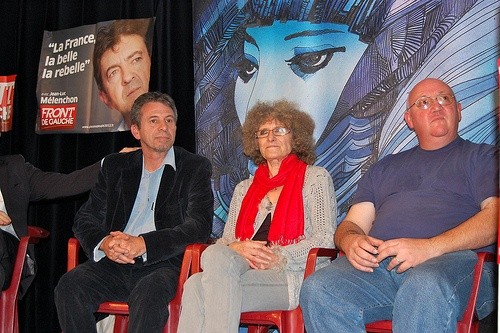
[394,257,400,263]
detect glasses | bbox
[407,94,456,113]
[254,127,291,138]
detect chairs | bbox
[0,226,497,333]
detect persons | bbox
[94,20,153,133]
[0,147,142,333]
[176,98,339,333]
[299,79,500,333]
[55,91,215,333]
